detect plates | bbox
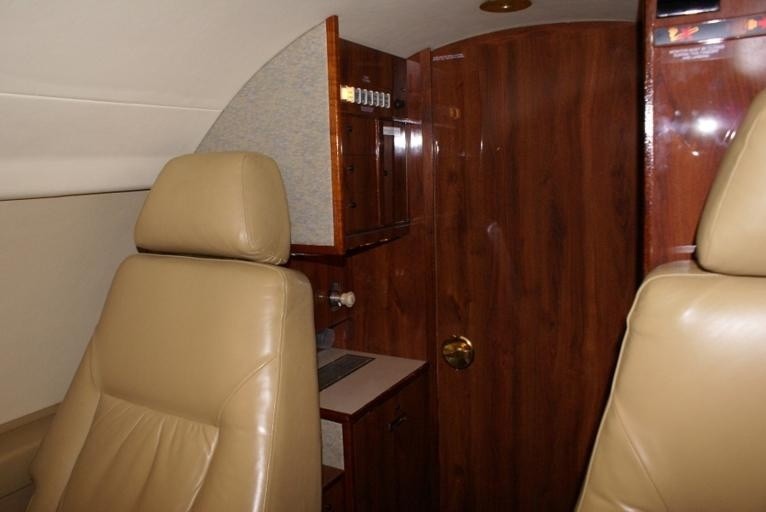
[317,366,427,509]
[194,38,408,258]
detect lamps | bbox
[567,84,765,511]
[26,151,323,510]
